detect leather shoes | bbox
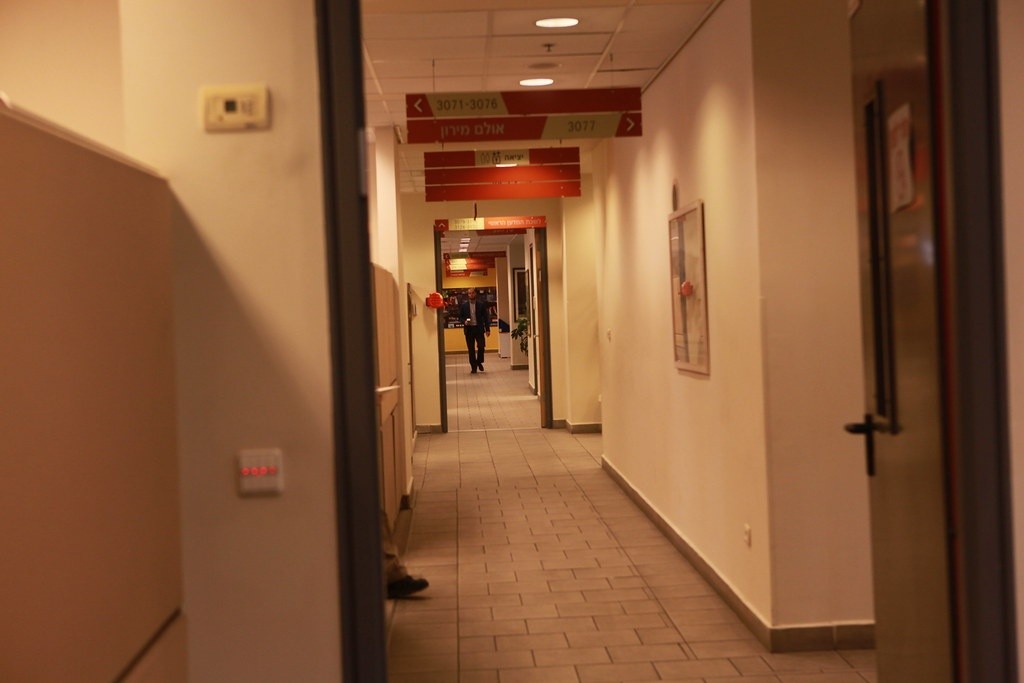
[471,367,477,372]
[389,574,430,599]
[476,359,485,371]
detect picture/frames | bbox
[668,201,712,378]
[512,268,528,324]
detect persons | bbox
[382,507,431,600]
[457,288,491,373]
